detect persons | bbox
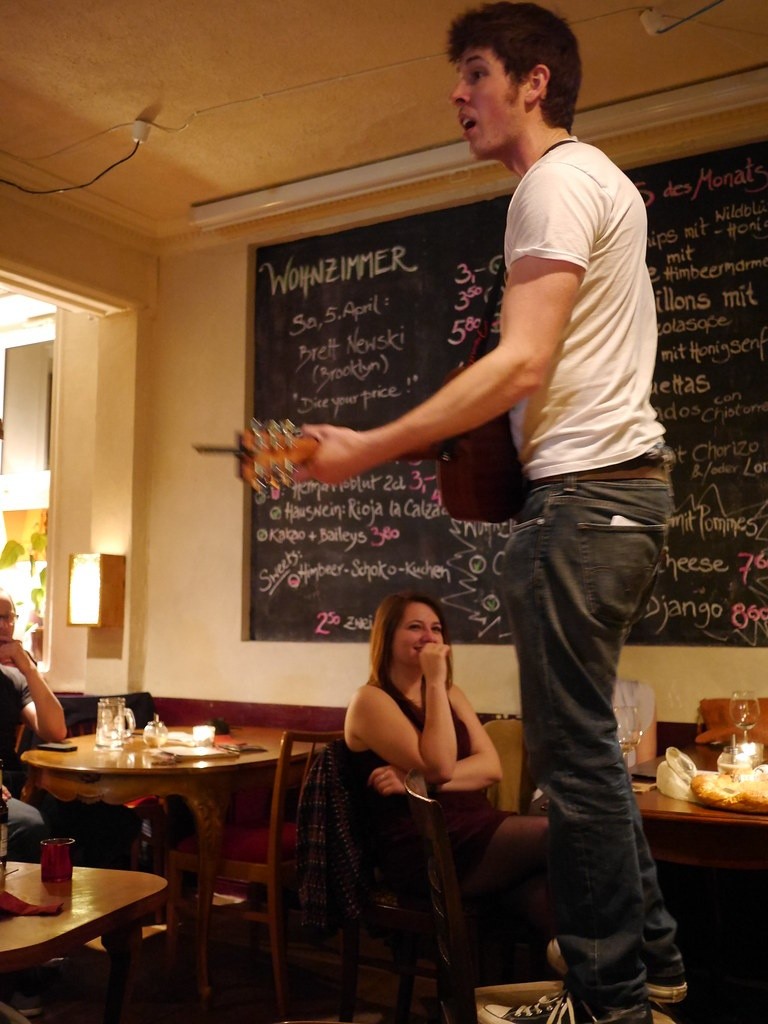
[342,592,561,978]
[0,598,69,864]
[295,1,689,1024]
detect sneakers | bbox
[546,938,688,1004]
[478,992,654,1024]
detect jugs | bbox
[143,714,169,746]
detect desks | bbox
[19,732,327,995]
[0,858,168,1023]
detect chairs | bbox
[166,730,441,1024]
[693,698,768,752]
[479,720,523,815]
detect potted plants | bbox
[0,532,48,660]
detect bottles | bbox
[717,748,752,776]
[0,758,9,863]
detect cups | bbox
[40,838,75,880]
[193,726,216,747]
[96,697,136,746]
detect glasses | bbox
[0,613,19,623]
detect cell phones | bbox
[37,742,78,752]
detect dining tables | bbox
[634,786,768,876]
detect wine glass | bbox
[730,691,760,744]
[611,706,642,766]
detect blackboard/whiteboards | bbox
[243,125,768,647]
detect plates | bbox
[160,746,236,758]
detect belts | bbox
[530,462,668,485]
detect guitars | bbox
[233,363,534,525]
